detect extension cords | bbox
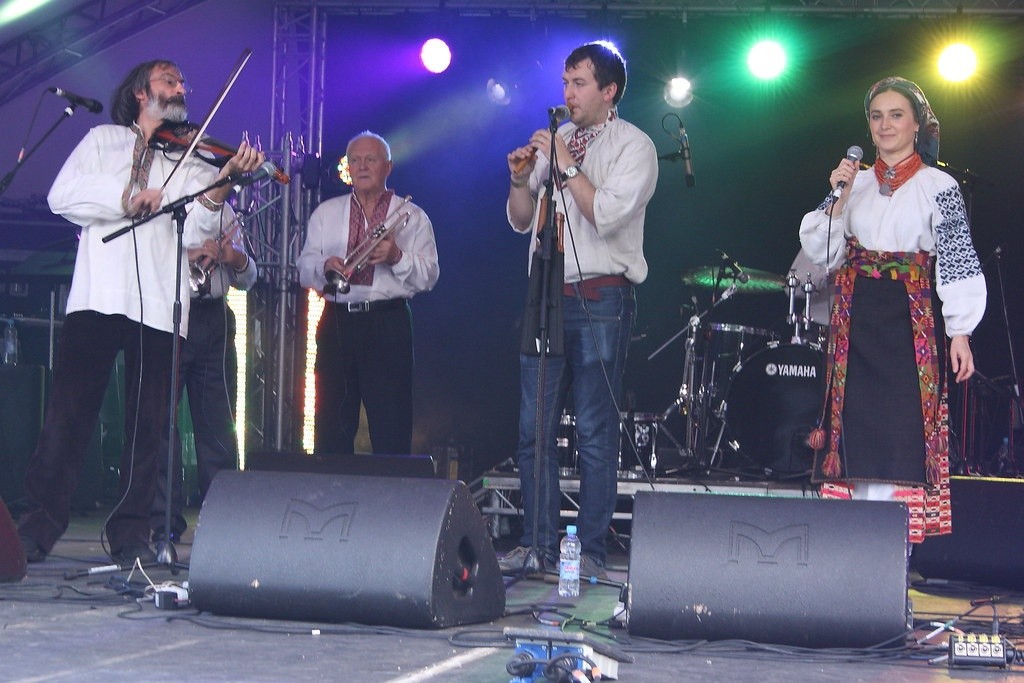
[152,586,189,607]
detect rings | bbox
[837,172,840,176]
[202,244,206,249]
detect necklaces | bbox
[873,150,922,197]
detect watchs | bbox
[561,163,582,182]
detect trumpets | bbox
[188,211,245,292]
[325,193,412,295]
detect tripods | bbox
[62,171,237,582]
[647,265,745,480]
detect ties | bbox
[551,108,619,191]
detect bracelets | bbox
[508,173,531,189]
[388,250,402,267]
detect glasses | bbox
[145,76,193,96]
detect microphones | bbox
[680,124,695,188]
[232,160,277,193]
[50,87,104,113]
[717,249,750,285]
[831,145,864,205]
[549,104,571,121]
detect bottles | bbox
[558,525,581,597]
[3,319,18,368]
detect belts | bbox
[562,275,632,301]
[191,297,223,304]
[324,299,409,314]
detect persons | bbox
[788,251,836,345]
[801,77,988,557]
[499,40,658,579]
[16,58,265,566]
[295,134,440,455]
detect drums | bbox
[693,321,784,392]
[717,339,829,481]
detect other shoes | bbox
[19,535,46,562]
[152,527,181,545]
[498,546,557,574]
[111,548,159,563]
[580,554,609,579]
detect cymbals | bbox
[678,265,788,293]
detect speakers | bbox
[627,490,913,649]
[188,468,507,629]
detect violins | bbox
[147,120,291,185]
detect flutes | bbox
[513,103,574,175]
